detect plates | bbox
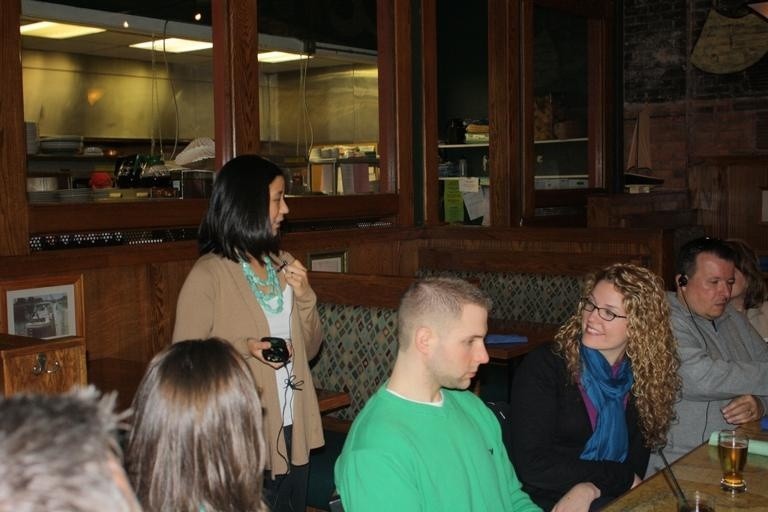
[26,188,116,205]
[24,121,39,156]
[36,134,84,156]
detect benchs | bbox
[395,250,646,326]
[305,271,423,437]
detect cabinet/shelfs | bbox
[414,0,621,226]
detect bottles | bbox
[307,147,370,196]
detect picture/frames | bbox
[2,272,86,340]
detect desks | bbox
[479,315,575,451]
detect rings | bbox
[290,271,294,278]
[745,408,753,416]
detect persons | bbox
[328,269,545,511]
[720,235,766,341]
[116,333,278,512]
[649,239,767,511]
[0,386,147,511]
[166,154,330,512]
[501,264,683,511]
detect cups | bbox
[717,430,749,494]
[677,489,716,512]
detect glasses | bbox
[579,296,627,321]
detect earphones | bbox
[678,276,687,288]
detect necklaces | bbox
[239,254,284,315]
